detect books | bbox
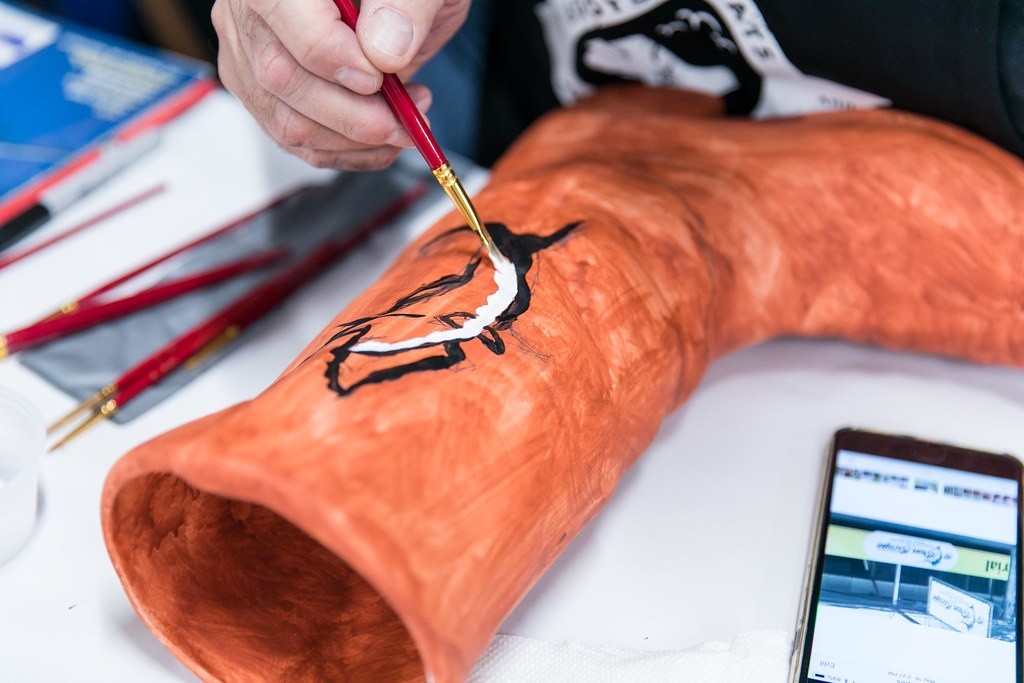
[0,0,217,271]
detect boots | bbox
[98,87,1024,683]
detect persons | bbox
[209,0,1024,176]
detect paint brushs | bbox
[334,0,509,272]
[0,172,432,467]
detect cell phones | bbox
[786,426,1023,683]
